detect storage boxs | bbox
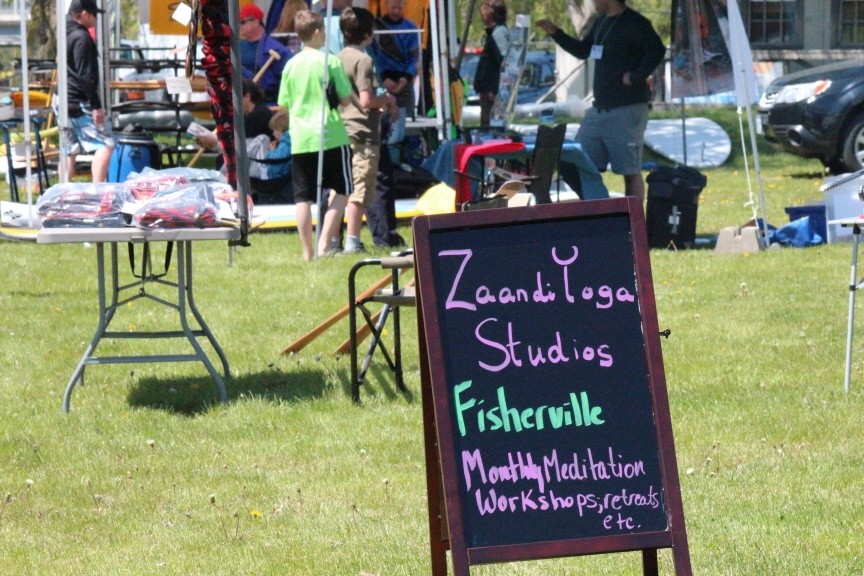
[784,201,830,244]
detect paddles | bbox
[188,48,282,167]
[280,263,415,356]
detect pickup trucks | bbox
[119,42,168,103]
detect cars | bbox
[756,53,864,175]
[456,47,557,106]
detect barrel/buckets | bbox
[107,138,160,183]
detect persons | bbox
[195,0,423,260]
[473,1,511,132]
[53,0,115,183]
[535,0,668,200]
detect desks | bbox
[36,223,242,411]
[422,136,611,214]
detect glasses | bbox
[239,18,254,24]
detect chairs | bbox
[106,57,203,166]
[454,123,568,204]
[349,194,508,407]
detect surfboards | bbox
[642,117,733,169]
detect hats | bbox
[68,0,106,14]
[240,4,264,20]
